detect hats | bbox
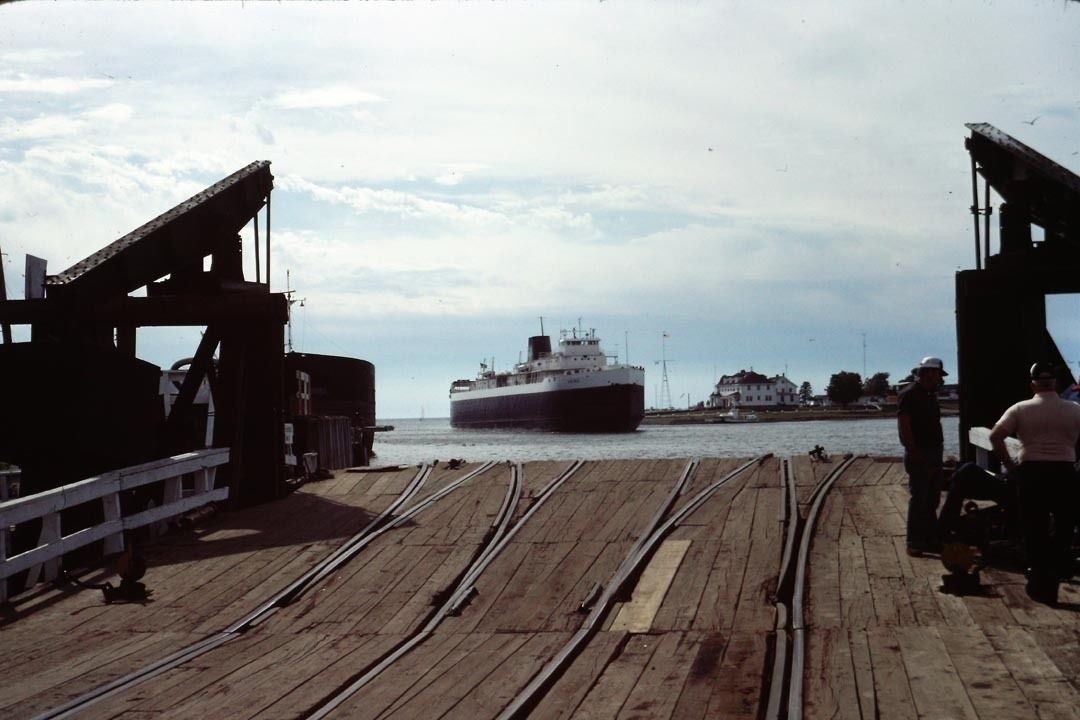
[915,356,949,377]
[1030,361,1058,380]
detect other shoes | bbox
[1025,582,1059,605]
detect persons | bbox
[897,356,950,556]
[989,362,1080,605]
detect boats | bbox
[448,315,646,433]
[711,408,760,424]
